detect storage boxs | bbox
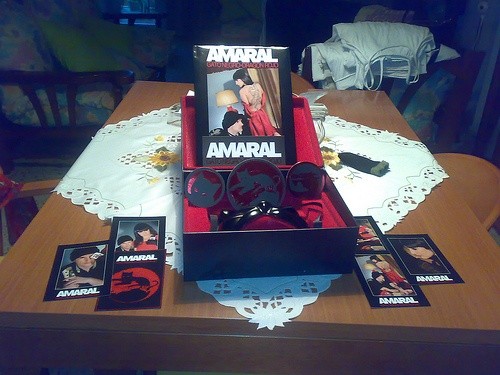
[180,95,360,281]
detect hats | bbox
[222,111,242,129]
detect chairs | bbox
[427,152,500,232]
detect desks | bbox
[0,80,500,375]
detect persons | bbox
[57,245,108,288]
[357,220,382,246]
[401,239,447,273]
[115,222,158,250]
[366,254,413,296]
[221,68,282,136]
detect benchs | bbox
[0,0,177,174]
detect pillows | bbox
[81,12,134,58]
[36,19,123,72]
